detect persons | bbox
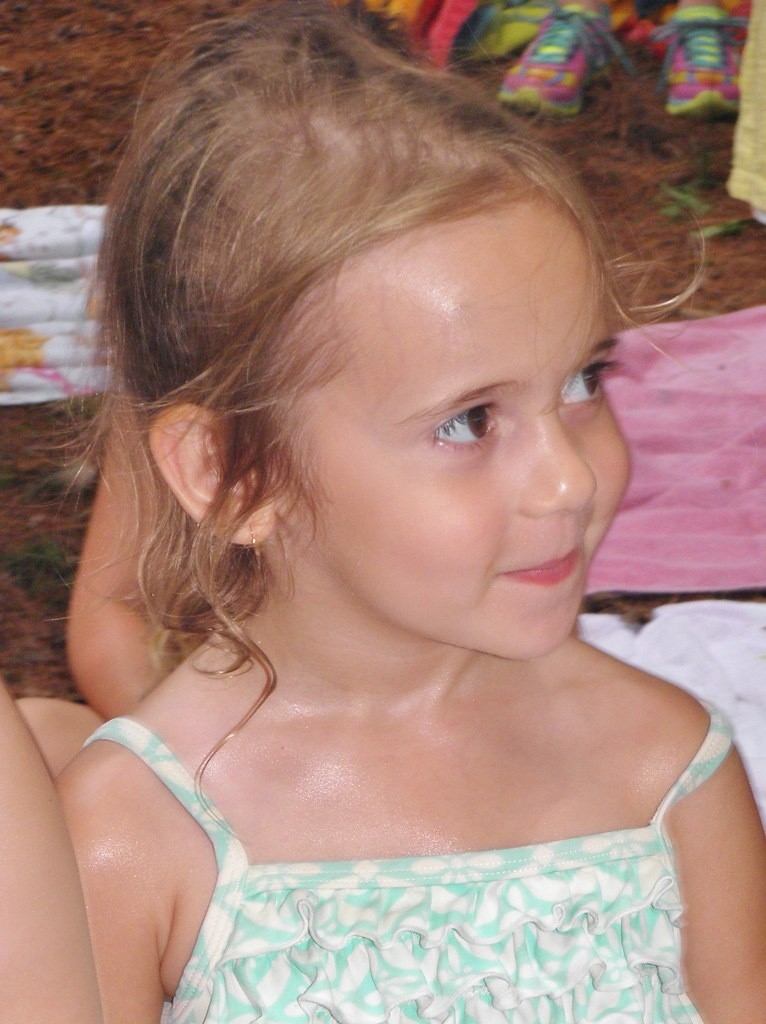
[497,0,742,117]
[0,0,766,1024]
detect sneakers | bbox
[646,15,750,121]
[494,3,638,117]
